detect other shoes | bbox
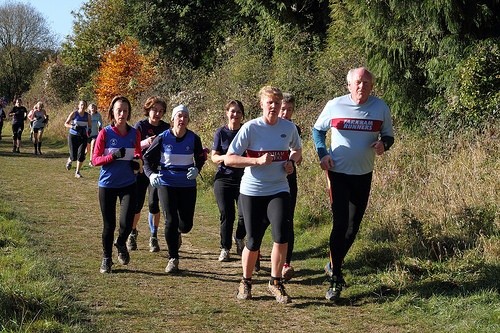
[34,151,42,155]
[13,148,20,153]
[89,161,94,167]
[66,159,82,178]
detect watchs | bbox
[288,159,297,169]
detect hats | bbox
[172,104,189,120]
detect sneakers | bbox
[149,236,160,252]
[237,280,252,300]
[165,258,179,273]
[179,233,182,247]
[234,236,245,256]
[128,230,139,251]
[218,248,230,262]
[100,257,114,273]
[254,251,262,272]
[114,237,130,265]
[282,263,294,282]
[268,280,290,304]
[325,260,346,300]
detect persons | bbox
[313,67,395,301]
[255,94,302,280]
[84,103,103,168]
[64,99,93,178]
[224,85,305,304]
[92,96,142,274]
[143,104,206,273]
[28,104,38,142]
[9,98,29,153]
[0,99,6,140]
[210,99,247,262]
[126,96,172,253]
[31,101,48,156]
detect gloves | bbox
[99,127,102,131]
[187,167,199,179]
[112,146,126,159]
[42,119,47,123]
[72,126,79,130]
[37,116,42,120]
[87,129,92,136]
[149,172,163,188]
[131,159,140,171]
